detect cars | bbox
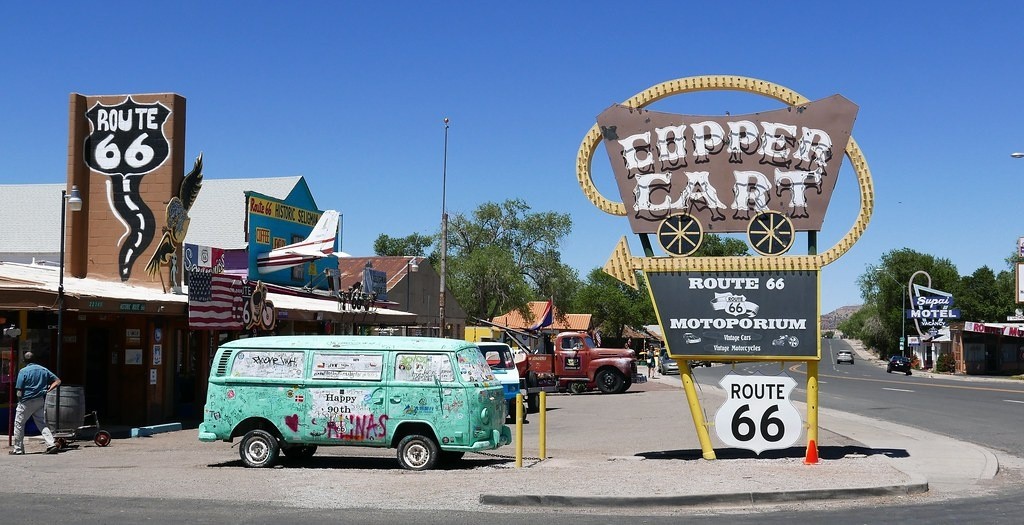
[886,356,912,375]
[836,349,855,364]
[660,352,691,375]
[691,360,712,368]
[655,347,666,373]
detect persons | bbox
[647,345,655,379]
[570,337,577,349]
[625,343,629,349]
[9,352,61,455]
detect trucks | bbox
[482,332,637,394]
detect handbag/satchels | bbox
[647,359,651,363]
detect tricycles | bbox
[53,410,112,451]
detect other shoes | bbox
[45,446,57,454]
[9,450,22,455]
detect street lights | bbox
[876,268,906,358]
[55,184,83,434]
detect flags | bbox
[595,330,601,347]
[531,298,553,329]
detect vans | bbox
[474,343,528,422]
[197,335,513,470]
